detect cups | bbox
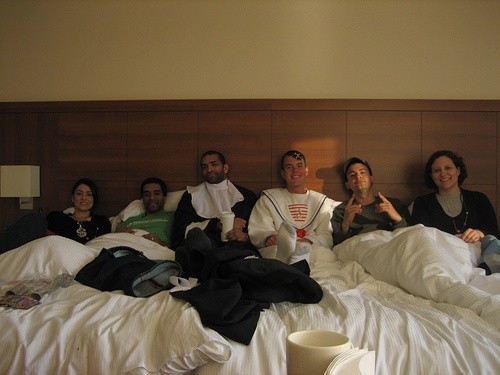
[285,329,354,375]
[220,212,235,241]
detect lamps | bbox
[0,165,41,210]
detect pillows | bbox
[117,189,187,223]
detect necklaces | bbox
[290,189,310,219]
[73,214,90,228]
[437,190,470,235]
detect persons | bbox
[0,207,77,258]
[247,149,334,266]
[330,157,413,247]
[411,149,500,276]
[175,150,261,275]
[114,177,177,251]
[65,179,113,246]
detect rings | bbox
[151,237,154,240]
